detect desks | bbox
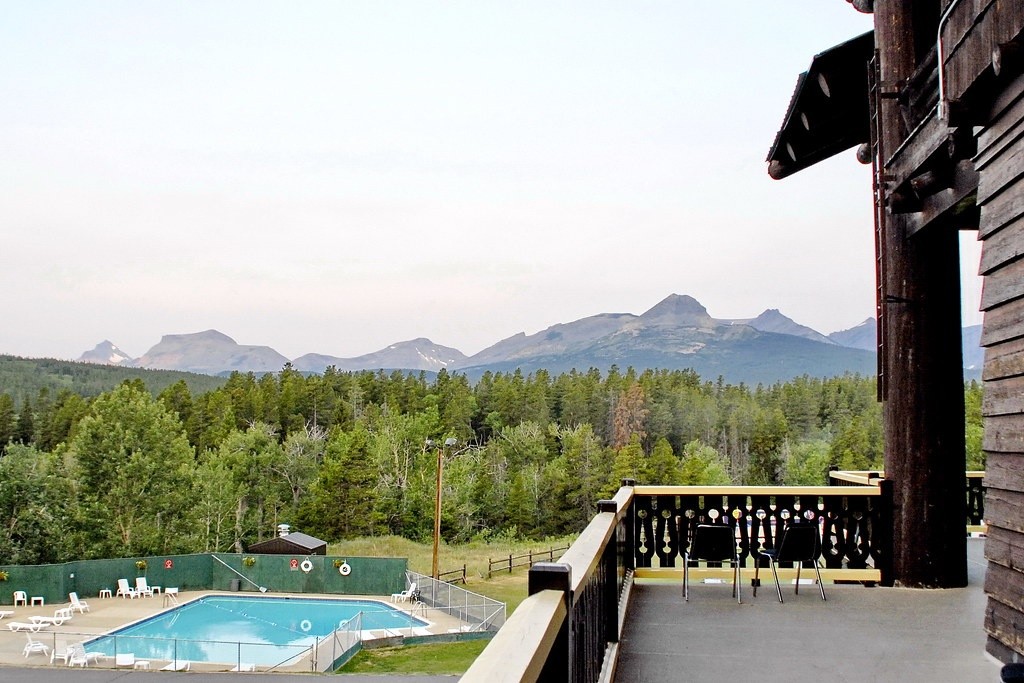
[151,585,161,595]
[100,590,112,600]
[55,608,73,617]
[134,660,150,669]
[31,596,44,607]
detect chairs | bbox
[355,624,486,640]
[753,522,826,603]
[28,615,72,626]
[160,659,191,670]
[231,663,256,671]
[0,591,27,619]
[165,588,178,597]
[117,579,138,599]
[391,582,421,603]
[22,631,49,658]
[51,639,107,667]
[6,622,51,633]
[135,576,153,598]
[682,525,742,603]
[116,653,135,667]
[68,592,89,614]
